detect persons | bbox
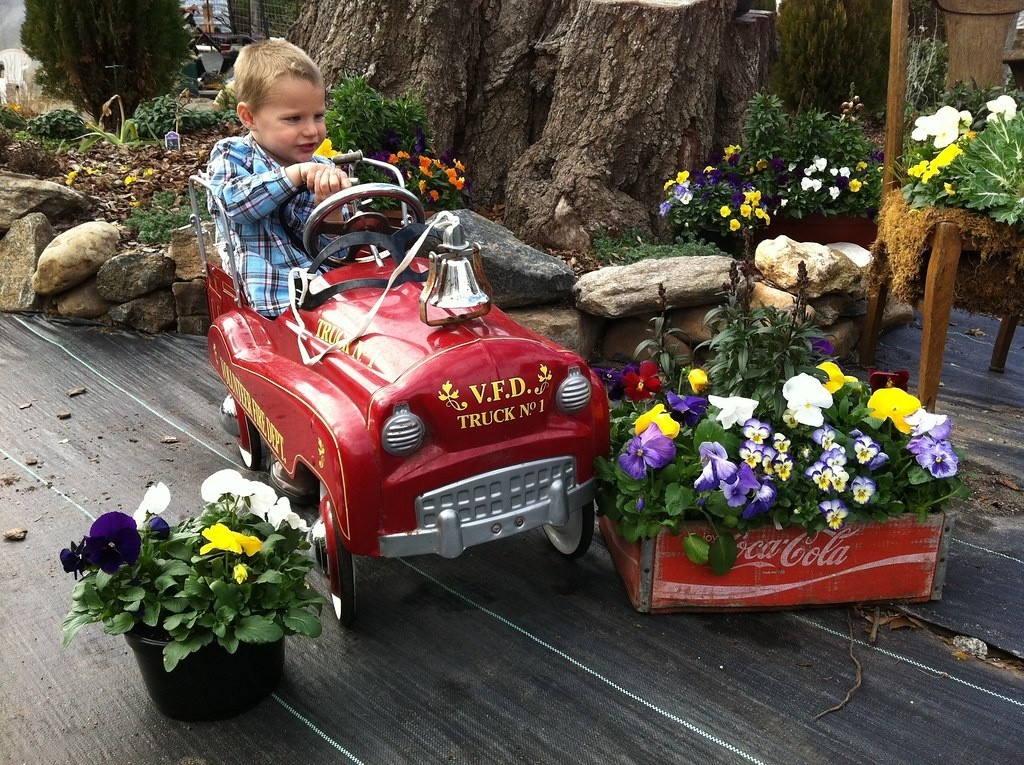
[206,39,352,321]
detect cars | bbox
[189,150,612,632]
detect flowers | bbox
[591,230,971,578]
[901,95,1024,225]
[60,468,326,674]
[313,73,466,209]
[658,83,884,232]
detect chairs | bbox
[861,0,1024,413]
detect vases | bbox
[383,210,403,236]
[124,607,285,720]
[750,207,878,259]
[418,211,436,224]
[600,482,957,614]
